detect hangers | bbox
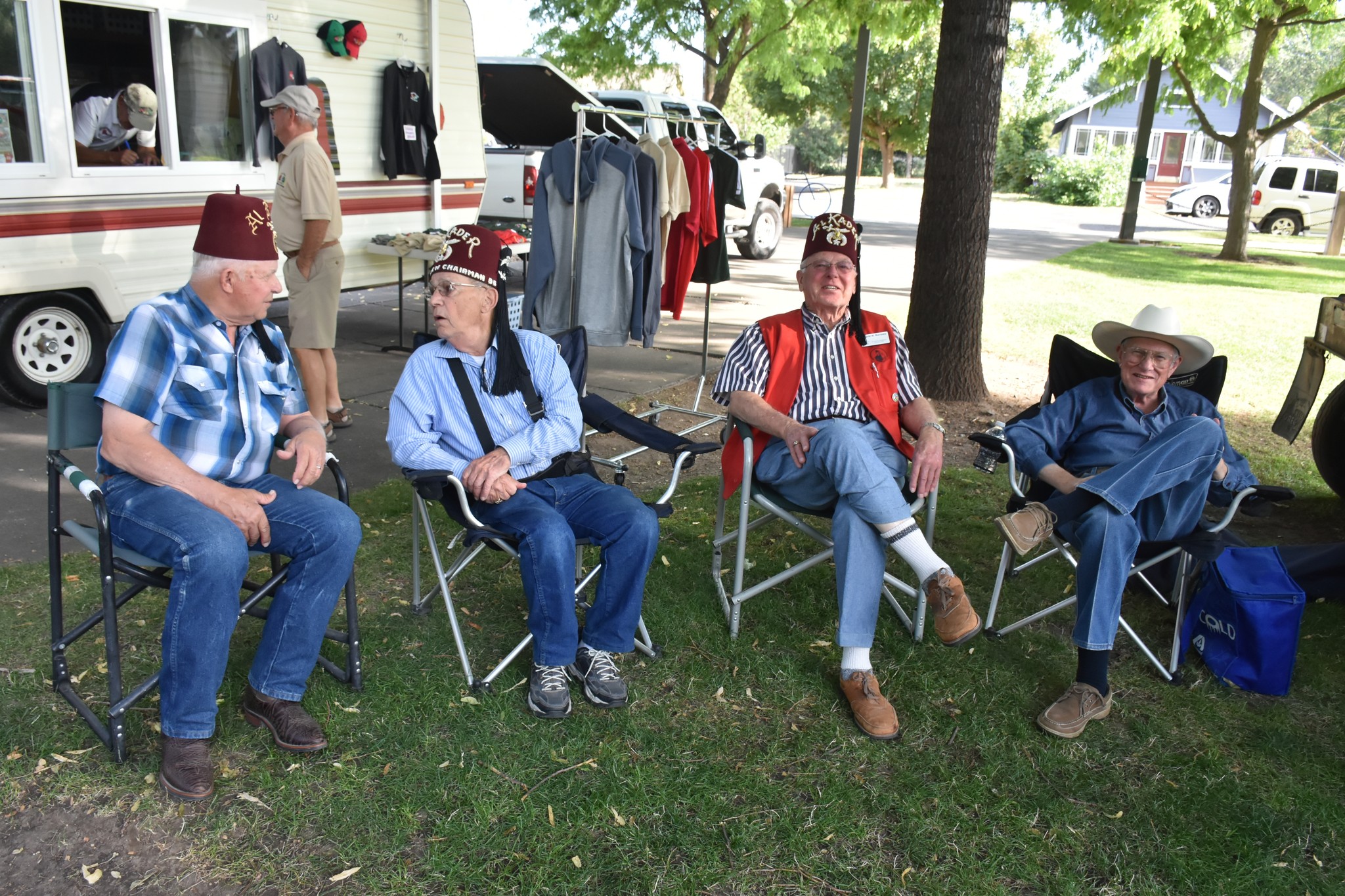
[684,115,698,147]
[687,116,715,146]
[398,40,413,63]
[569,103,599,141]
[591,106,621,140]
[275,19,283,42]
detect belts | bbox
[283,239,341,259]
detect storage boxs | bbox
[505,292,525,330]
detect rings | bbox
[316,466,321,470]
[494,498,503,504]
[792,440,801,447]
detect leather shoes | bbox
[994,499,1058,556]
[1037,679,1114,737]
[242,686,328,753]
[156,729,213,800]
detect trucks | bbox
[2,0,489,411]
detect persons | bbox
[996,319,1258,740]
[269,85,354,442]
[384,245,660,719]
[95,227,364,800]
[71,83,173,164]
[727,228,980,739]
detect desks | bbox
[367,242,531,353]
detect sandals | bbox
[324,422,337,442]
[327,407,353,428]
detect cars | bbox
[1165,172,1233,218]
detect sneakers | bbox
[568,646,629,709]
[926,566,982,647]
[528,660,573,720]
[837,672,899,739]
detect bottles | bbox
[974,421,1006,474]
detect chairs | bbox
[46,382,363,764]
[968,335,1295,684]
[711,410,940,644]
[403,327,722,695]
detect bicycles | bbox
[784,170,832,217]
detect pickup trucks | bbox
[474,53,786,273]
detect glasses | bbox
[801,260,856,274]
[1122,344,1179,369]
[422,280,488,301]
[268,107,299,117]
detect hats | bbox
[431,223,525,398]
[260,85,322,118]
[317,19,348,58]
[124,83,157,131]
[805,212,867,347]
[193,186,285,364]
[342,20,367,61]
[1091,304,1214,373]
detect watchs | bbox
[919,423,946,441]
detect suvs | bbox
[1249,155,1345,238]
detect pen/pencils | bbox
[872,362,880,378]
[124,140,131,149]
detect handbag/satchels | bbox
[1182,544,1305,698]
